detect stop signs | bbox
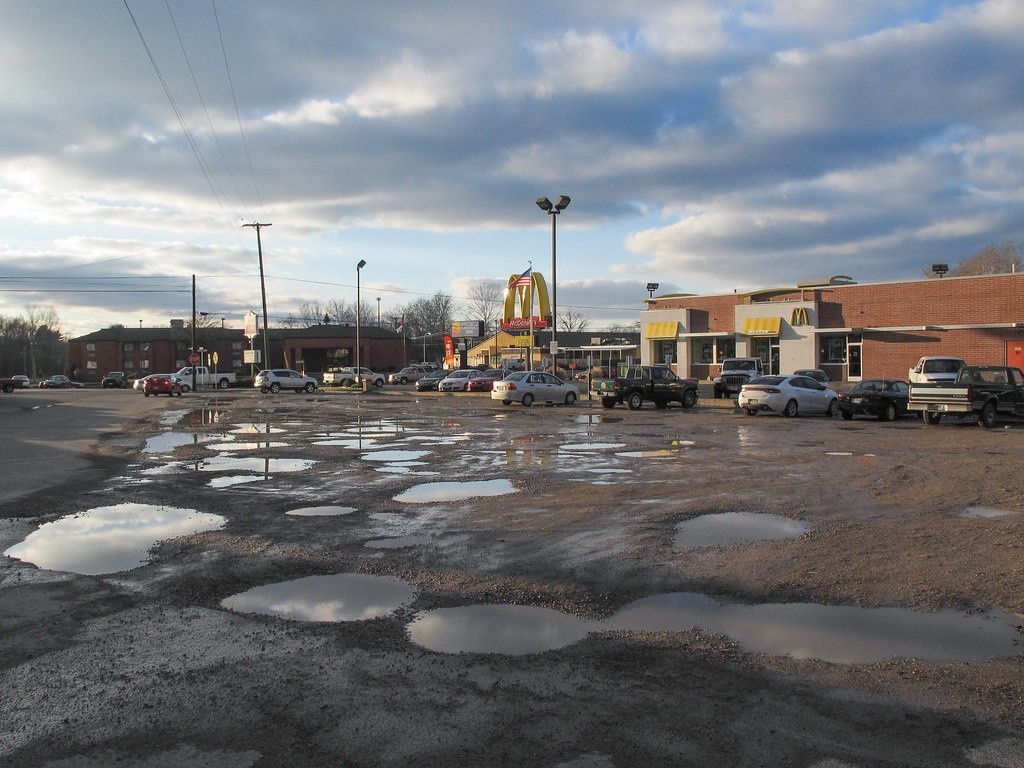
[189,353,201,366]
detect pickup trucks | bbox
[906,365,1024,427]
[909,356,968,383]
[322,367,386,388]
[174,367,237,392]
[0,379,23,393]
[591,366,700,409]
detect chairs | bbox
[992,374,1005,383]
[868,386,875,390]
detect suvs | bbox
[713,357,763,398]
[254,369,319,394]
[388,366,433,385]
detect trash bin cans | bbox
[362,379,372,392]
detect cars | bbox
[491,371,580,405]
[738,375,839,418]
[11,375,30,388]
[438,369,485,391]
[793,369,833,390]
[414,370,454,392]
[44,374,73,388]
[101,371,182,397]
[467,369,515,392]
[838,378,923,422]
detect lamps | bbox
[932,264,948,277]
[647,283,659,297]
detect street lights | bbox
[376,297,382,328]
[350,259,367,388]
[535,194,572,381]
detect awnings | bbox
[741,317,781,337]
[644,321,679,341]
